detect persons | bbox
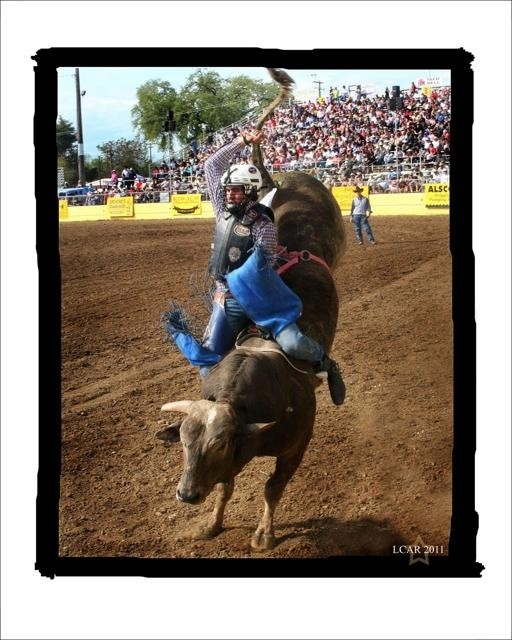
[59,81,450,206]
[199,127,346,405]
[350,186,377,246]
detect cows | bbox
[154,66,348,553]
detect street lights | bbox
[389,86,403,192]
[162,110,176,195]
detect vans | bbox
[59,187,96,204]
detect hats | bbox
[353,186,363,193]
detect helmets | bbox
[221,165,262,195]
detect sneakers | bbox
[327,360,346,405]
